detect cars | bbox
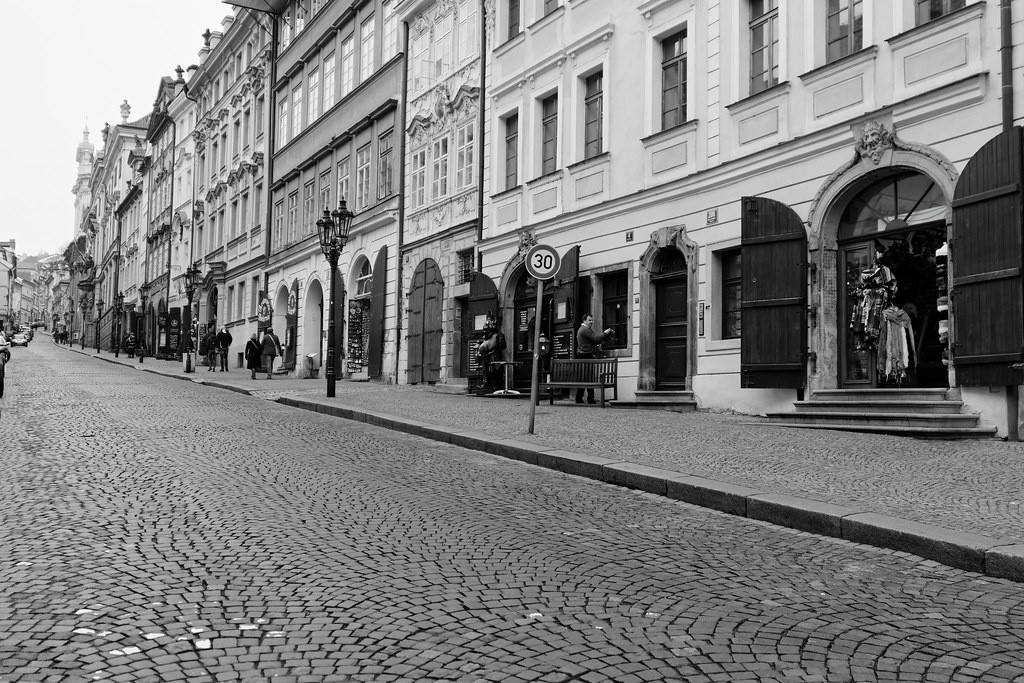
[0,350,6,397]
[0,334,11,363]
[9,333,28,347]
[0,320,45,342]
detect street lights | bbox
[63,311,68,345]
[138,281,151,363]
[81,301,87,350]
[96,299,106,353]
[181,262,202,373]
[114,291,125,357]
[70,308,75,347]
[315,195,353,398]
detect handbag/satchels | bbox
[275,345,279,356]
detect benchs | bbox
[536,357,617,408]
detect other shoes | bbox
[220,369,224,371]
[213,368,215,372]
[251,376,256,379]
[225,367,228,371]
[208,368,212,371]
[266,375,271,379]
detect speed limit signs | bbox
[525,243,561,280]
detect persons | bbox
[261,328,283,379]
[125,332,136,358]
[575,313,611,404]
[51,327,69,345]
[476,320,507,390]
[245,333,261,379]
[205,328,217,372]
[217,327,233,372]
